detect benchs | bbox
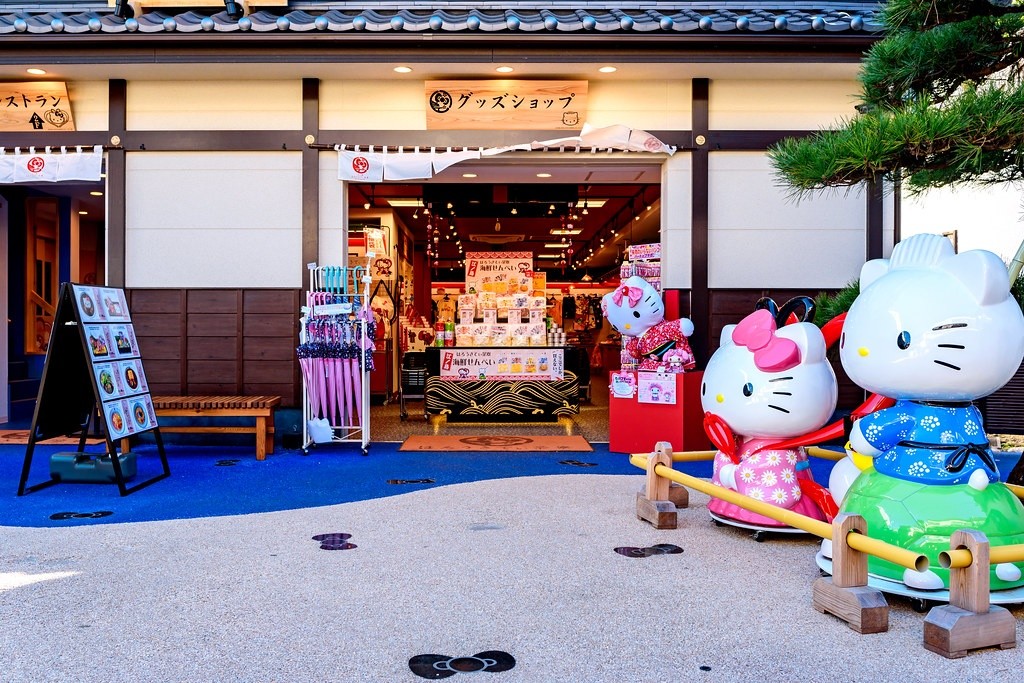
[105,394,282,461]
[425,369,580,423]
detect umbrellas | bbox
[296,264,376,428]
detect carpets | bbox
[398,435,594,452]
[0,429,107,444]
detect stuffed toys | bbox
[820,233,1024,592]
[701,296,839,533]
[600,275,697,373]
[663,348,689,373]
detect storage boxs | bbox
[455,309,546,348]
[607,370,710,455]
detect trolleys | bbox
[399,350,429,422]
